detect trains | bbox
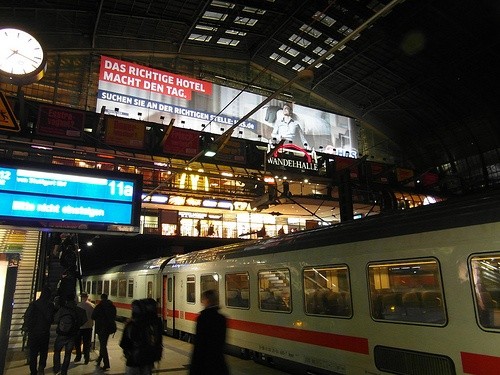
[80,188,500,375]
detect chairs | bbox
[228,290,498,327]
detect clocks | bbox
[0,24,48,86]
